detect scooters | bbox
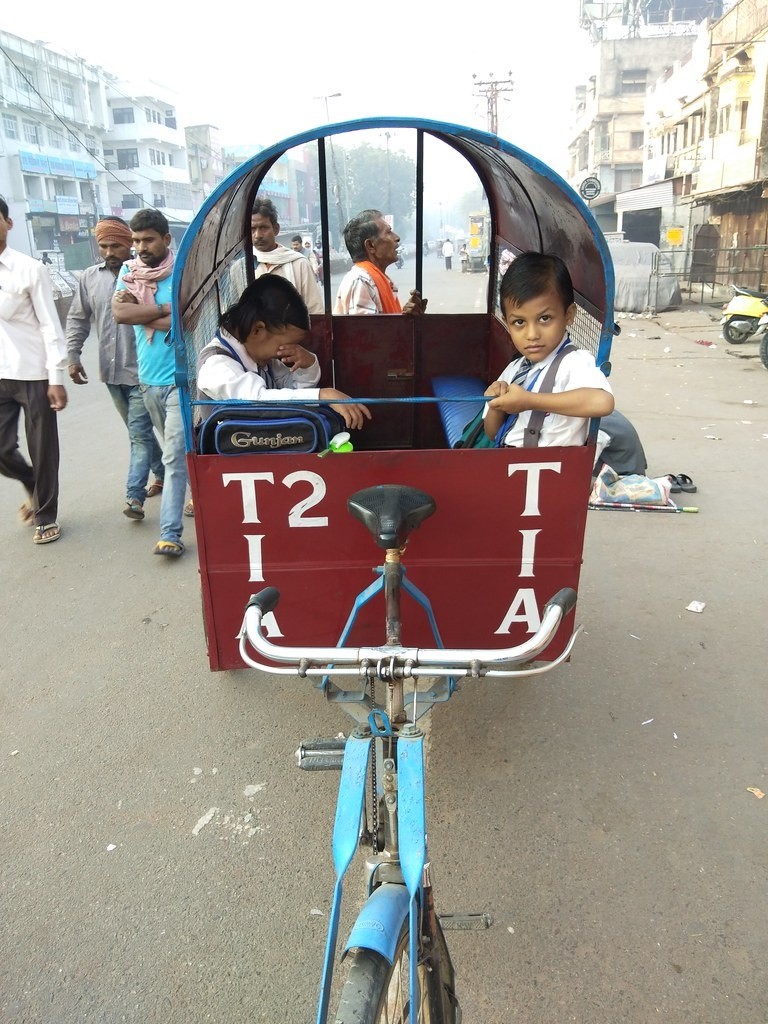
[720,284,768,370]
[393,248,405,269]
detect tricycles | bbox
[436,245,444,258]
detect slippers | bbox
[18,496,36,528]
[182,499,195,516]
[144,480,163,497]
[152,538,185,556]
[674,474,696,493]
[32,520,60,544]
[123,503,145,521]
[656,474,681,493]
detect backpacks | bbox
[193,403,345,455]
[452,406,498,448]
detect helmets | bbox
[315,239,322,248]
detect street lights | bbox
[325,93,348,253]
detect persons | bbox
[111,208,196,555]
[64,217,165,519]
[484,255,490,271]
[42,252,52,265]
[458,244,468,263]
[395,242,404,262]
[333,209,427,315]
[591,405,647,477]
[481,250,614,448]
[423,238,453,252]
[252,198,325,314]
[291,235,324,285]
[195,273,372,430]
[442,239,453,271]
[0,195,68,543]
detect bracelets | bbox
[158,304,163,315]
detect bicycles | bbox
[170,117,619,1024]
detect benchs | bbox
[429,374,489,450]
